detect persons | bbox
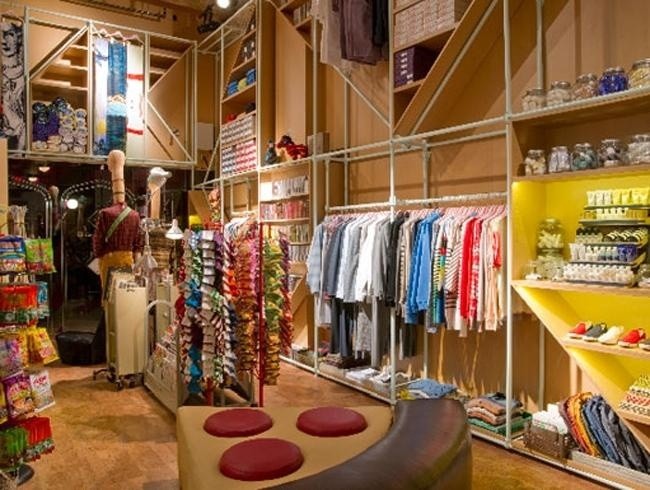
[90,148,142,356]
[143,164,179,302]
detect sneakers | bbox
[565,320,650,351]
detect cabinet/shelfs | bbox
[509,82,650,489]
[103,219,287,420]
[22,5,200,165]
[259,160,348,351]
[270,1,518,136]
[217,0,275,179]
[1,219,36,489]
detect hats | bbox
[148,166,171,179]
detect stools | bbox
[172,396,476,489]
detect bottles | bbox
[522,58,650,289]
